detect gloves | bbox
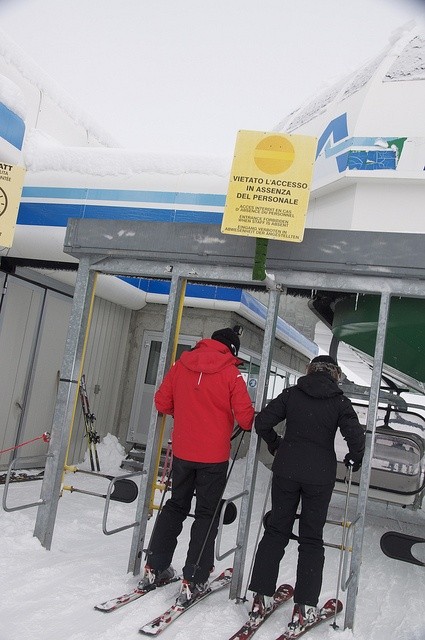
[342,452,363,472]
[267,436,282,457]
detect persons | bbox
[247,356,366,635]
[142,328,255,604]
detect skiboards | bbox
[78,374,103,471]
[230,584,345,640]
[93,558,235,635]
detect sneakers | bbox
[143,566,175,584]
[291,604,320,628]
[180,580,210,598]
[251,595,277,622]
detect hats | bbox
[211,326,240,356]
[307,355,338,381]
